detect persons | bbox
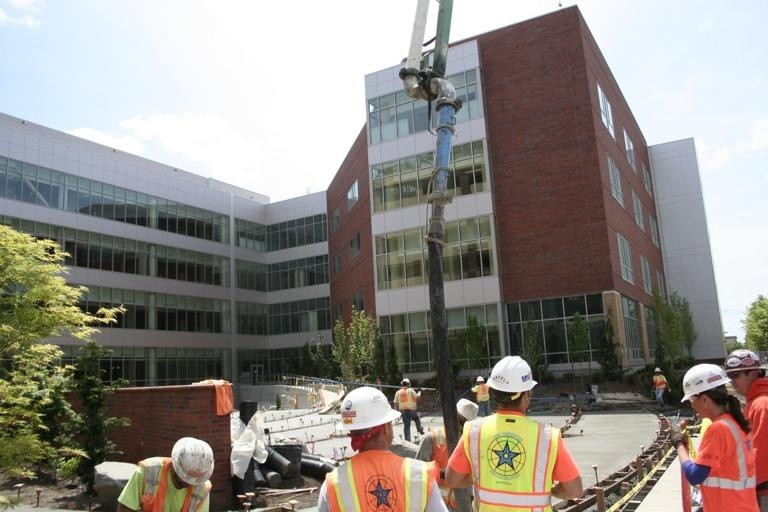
[316,385,449,511]
[470,375,494,417]
[651,366,672,411]
[670,346,768,512]
[445,354,585,511]
[116,435,215,512]
[392,377,425,444]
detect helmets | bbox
[681,364,731,402]
[457,399,479,420]
[724,350,768,373]
[476,377,484,381]
[487,356,538,393]
[341,387,401,430]
[171,437,215,485]
[655,368,661,372]
[401,379,410,386]
